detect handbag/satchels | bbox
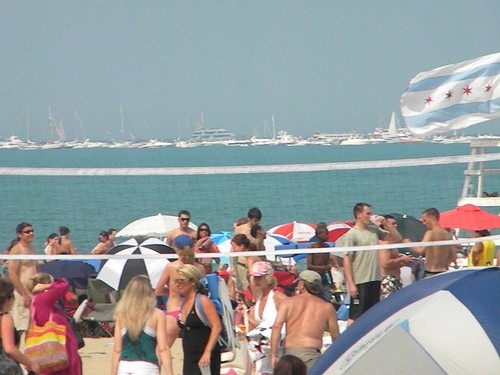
[0,354,23,375]
[24,321,68,375]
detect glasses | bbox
[175,279,185,284]
[24,230,33,233]
[199,229,208,231]
[181,218,190,222]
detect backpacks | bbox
[181,274,234,352]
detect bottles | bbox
[369,215,381,226]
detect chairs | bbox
[70,276,119,338]
[201,275,240,364]
[274,241,335,278]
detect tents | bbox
[307,265,500,375]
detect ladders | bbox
[450,137,500,267]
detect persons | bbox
[270,270,341,371]
[468,229,495,266]
[111,275,173,375]
[378,215,413,299]
[0,222,85,375]
[93,229,118,254]
[154,210,286,375]
[344,203,402,329]
[274,355,306,375]
[307,227,334,287]
[403,208,456,279]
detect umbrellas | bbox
[38,259,103,278]
[265,234,292,245]
[96,237,178,292]
[267,221,318,241]
[420,204,500,230]
[389,213,426,242]
[210,231,232,263]
[326,221,355,242]
[115,213,198,238]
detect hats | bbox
[293,270,322,285]
[247,262,273,276]
[173,234,194,249]
[16,222,31,241]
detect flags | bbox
[400,52,500,138]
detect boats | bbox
[455,135,500,217]
[0,111,494,151]
